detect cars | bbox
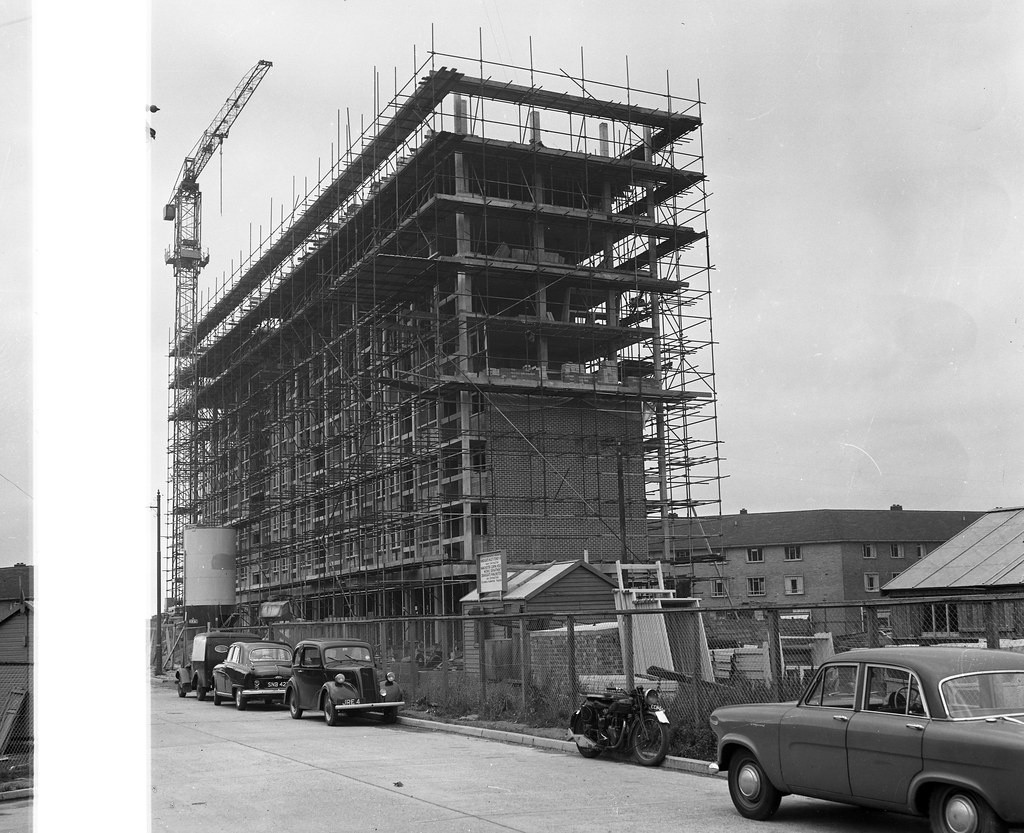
[705,645,1024,833]
[283,637,407,727]
[174,632,262,701]
[209,641,294,712]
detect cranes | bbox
[162,57,274,619]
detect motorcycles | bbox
[565,686,672,768]
